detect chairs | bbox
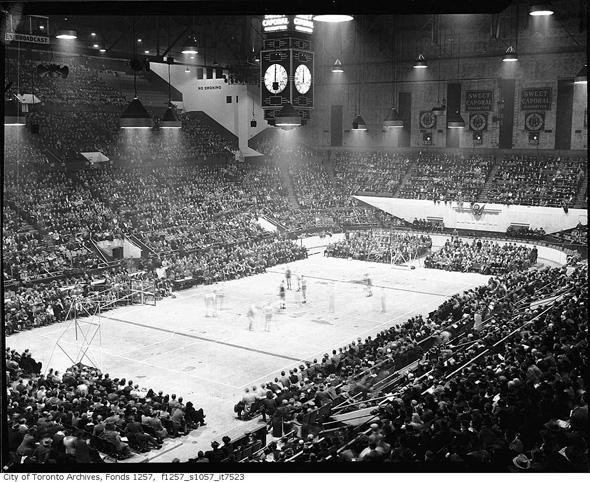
[0,117,587,462]
[0,45,167,117]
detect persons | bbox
[1,53,129,467]
[129,113,589,468]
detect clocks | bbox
[293,60,315,96]
[262,60,287,100]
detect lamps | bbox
[528,0,555,17]
[571,0,589,85]
[351,0,370,134]
[446,0,470,133]
[176,16,201,59]
[117,1,155,130]
[271,101,304,130]
[159,0,185,129]
[329,52,346,78]
[379,0,404,132]
[411,46,429,72]
[53,16,81,44]
[501,0,519,63]
[432,27,444,112]
